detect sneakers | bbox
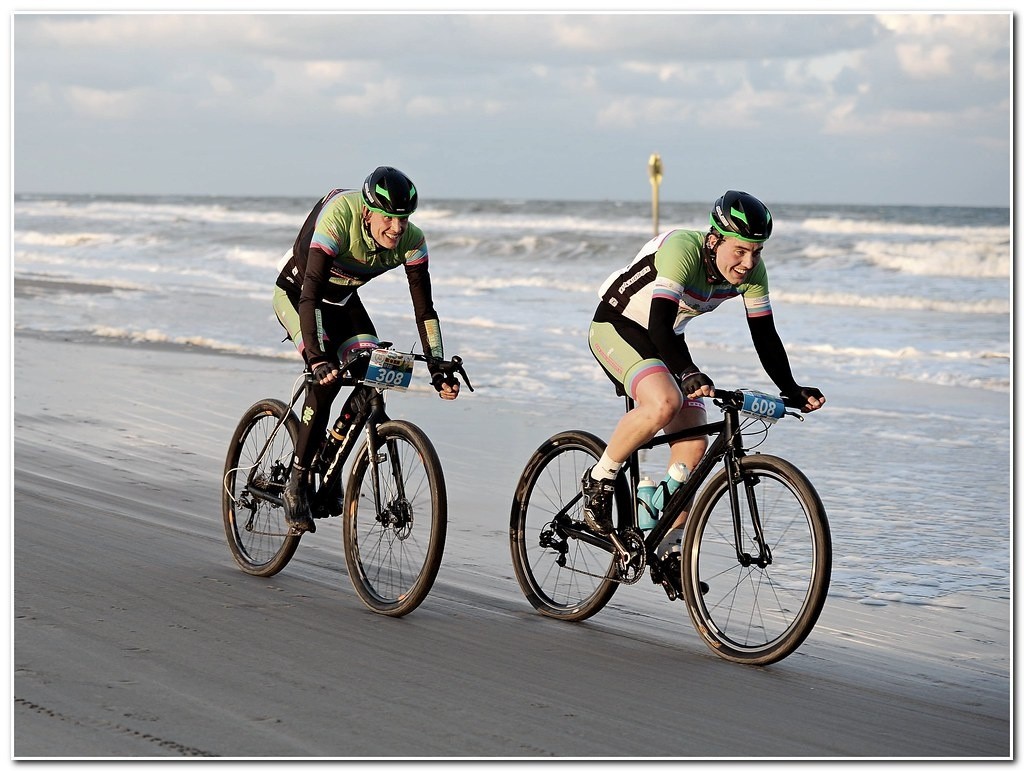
[650,551,709,595]
[282,483,316,533]
[581,464,615,534]
[316,450,344,516]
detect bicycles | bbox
[508,384,836,667]
[220,341,475,618]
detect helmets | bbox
[360,166,418,217]
[710,190,773,243]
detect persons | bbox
[581,189,827,601]
[272,166,460,533]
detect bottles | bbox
[320,413,350,464]
[651,462,689,510]
[636,477,660,531]
